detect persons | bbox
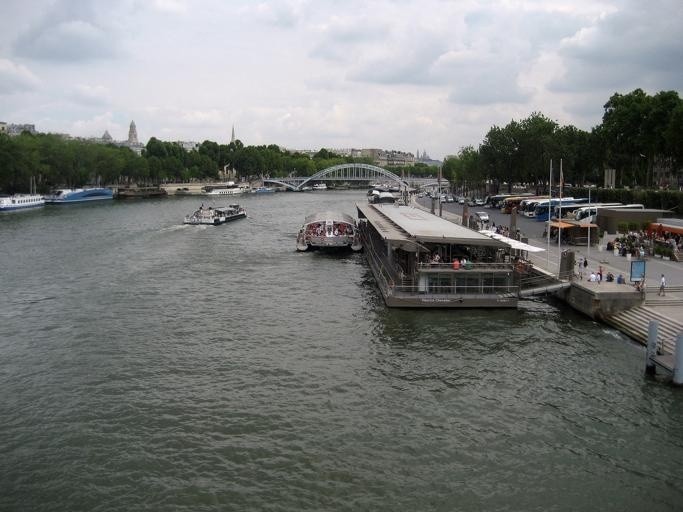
[194,206,202,221]
[483,221,681,296]
[432,251,473,270]
[209,207,215,219]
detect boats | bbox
[302,183,360,191]
[356,202,519,310]
[184,204,247,226]
[297,212,361,253]
[45,187,113,203]
[118,187,167,199]
[201,184,251,195]
[367,183,402,204]
[250,186,275,193]
[0,193,46,212]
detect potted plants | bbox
[653,245,673,260]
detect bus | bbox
[485,195,644,225]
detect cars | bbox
[419,191,484,207]
[475,212,489,223]
